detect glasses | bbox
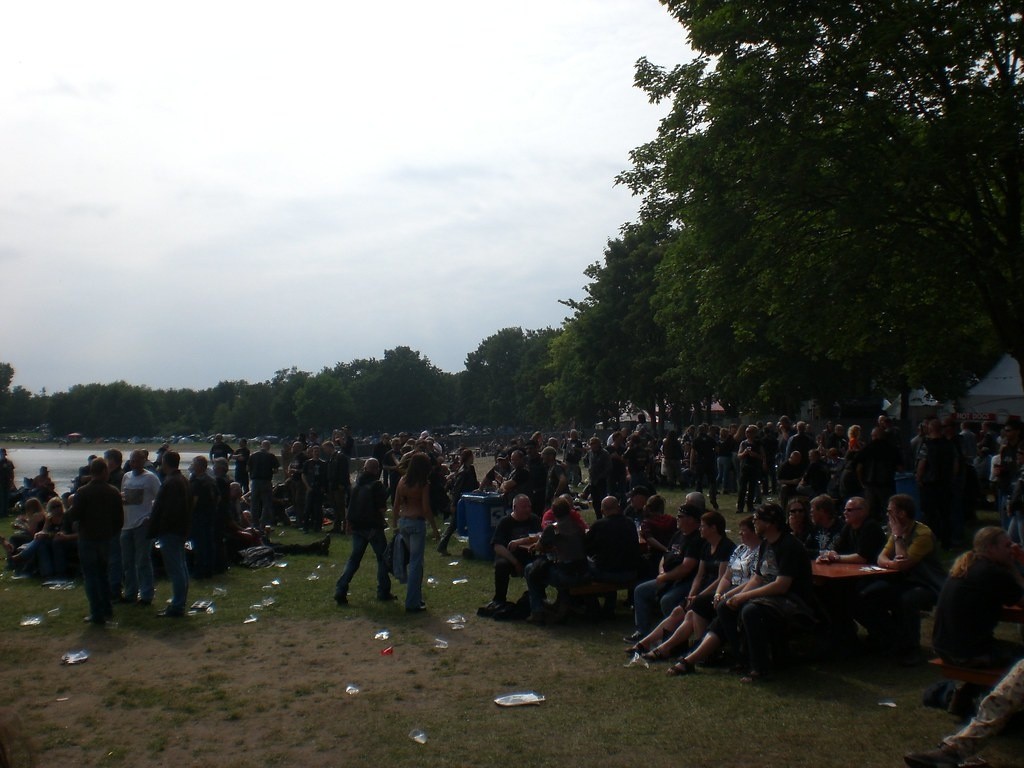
[790,509,806,514]
[1004,428,1017,432]
[843,508,866,512]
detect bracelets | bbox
[722,594,726,600]
[659,572,663,574]
[838,555,841,561]
[893,535,903,541]
[714,594,721,597]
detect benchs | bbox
[569,580,639,595]
[928,658,1009,687]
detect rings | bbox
[509,542,511,544]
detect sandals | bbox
[741,669,768,682]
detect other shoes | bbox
[622,630,695,676]
[379,591,398,600]
[901,647,921,666]
[710,500,718,508]
[406,599,427,614]
[84,616,92,621]
[487,596,545,626]
[12,553,27,560]
[904,741,963,768]
[156,607,168,617]
[437,545,451,556]
[737,509,743,513]
[320,534,330,554]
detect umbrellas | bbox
[65,432,81,437]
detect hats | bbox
[419,430,429,440]
[497,452,508,460]
[628,486,648,496]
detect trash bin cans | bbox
[454,492,504,561]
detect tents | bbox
[953,351,1024,423]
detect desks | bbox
[895,472,921,521]
[810,559,900,586]
[518,521,648,564]
[999,605,1024,624]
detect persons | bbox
[0,413,1024,767]
